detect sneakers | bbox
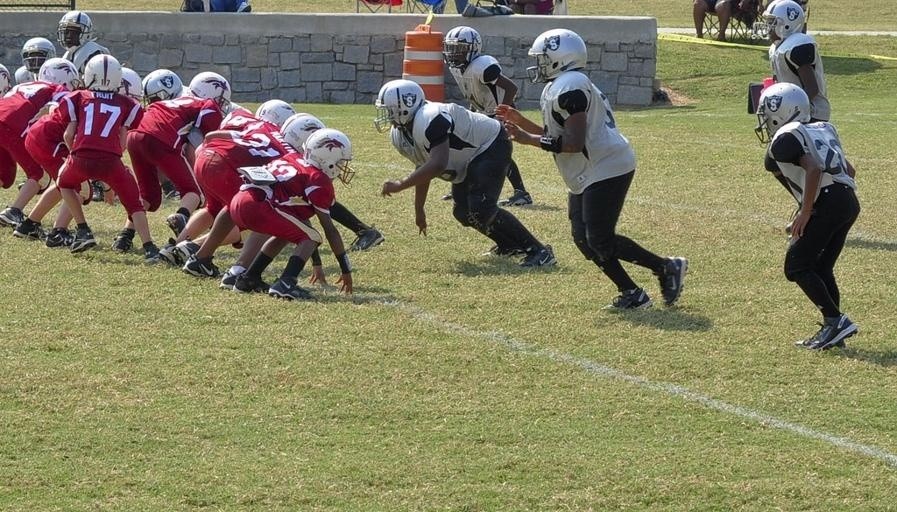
[159,242,183,267]
[345,228,385,253]
[11,221,46,240]
[267,278,318,301]
[45,231,74,248]
[480,244,523,258]
[232,273,269,294]
[165,212,188,238]
[793,324,845,349]
[517,245,558,268]
[440,190,452,199]
[177,239,201,263]
[0,207,26,227]
[181,254,221,279]
[496,190,532,206]
[219,268,246,289]
[88,183,103,201]
[652,254,688,308]
[142,245,162,263]
[597,288,652,312]
[806,314,859,350]
[67,228,96,254]
[109,235,133,253]
[161,181,176,199]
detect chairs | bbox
[703,0,758,42]
[406,0,446,14]
[357,0,402,14]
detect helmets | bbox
[523,28,588,86]
[0,64,11,97]
[371,78,426,135]
[19,37,56,73]
[279,112,325,154]
[54,11,93,51]
[300,128,354,186]
[751,82,813,142]
[751,0,806,41]
[142,69,184,107]
[121,67,143,101]
[84,54,121,93]
[255,100,295,128]
[188,71,231,116]
[440,24,483,71]
[38,58,83,92]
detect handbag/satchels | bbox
[179,0,252,12]
[462,0,515,17]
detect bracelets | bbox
[539,135,562,157]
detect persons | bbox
[0,11,384,303]
[373,80,557,269]
[443,27,533,204]
[694,0,731,41]
[507,1,550,14]
[493,29,688,313]
[756,81,861,351]
[763,0,832,121]
